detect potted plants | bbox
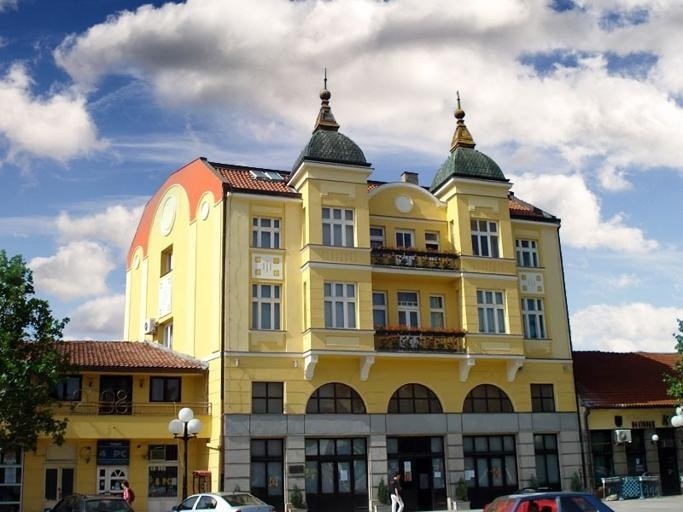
[452,477,471,512]
[373,477,391,512]
[287,484,309,512]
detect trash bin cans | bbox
[602,477,623,499]
[640,476,659,498]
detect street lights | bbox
[168,406,201,500]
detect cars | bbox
[44,493,134,512]
[171,491,274,511]
[484,491,615,512]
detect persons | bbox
[390,473,405,512]
[122,480,132,505]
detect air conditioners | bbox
[613,429,632,443]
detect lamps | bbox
[649,434,658,446]
